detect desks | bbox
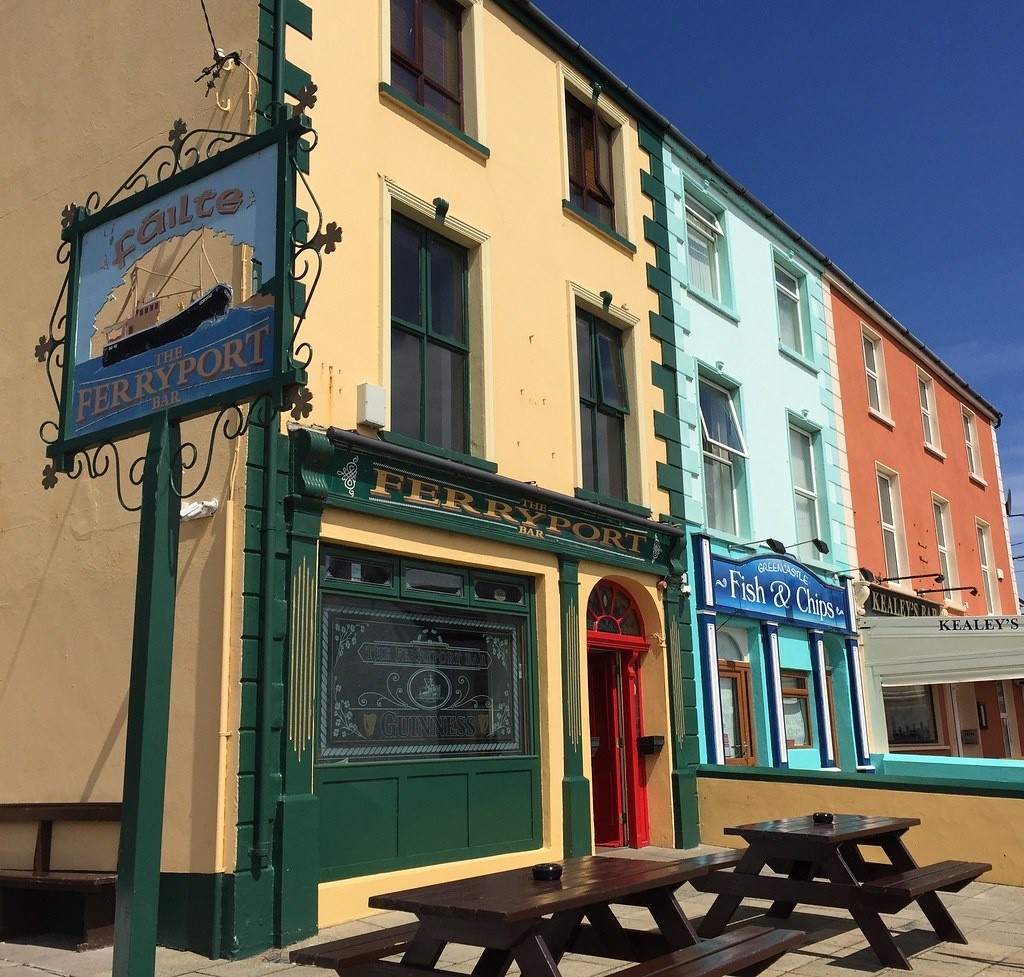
[335,855,789,977]
[686,813,984,971]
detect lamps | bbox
[785,538,830,555]
[877,573,944,586]
[826,566,875,583]
[917,586,978,597]
[729,539,786,555]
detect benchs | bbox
[671,848,749,873]
[0,801,122,953]
[862,861,992,899]
[289,921,423,970]
[602,926,807,977]
